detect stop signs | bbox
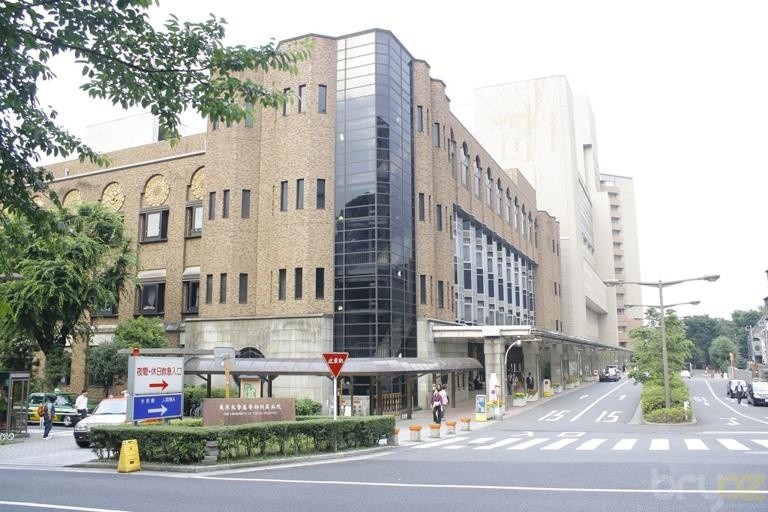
[323,353,349,378]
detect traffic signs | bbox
[127,354,185,395]
[126,392,185,423]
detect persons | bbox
[473,376,481,399]
[2,385,14,429]
[74,389,88,420]
[734,381,743,404]
[430,383,448,425]
[43,395,57,439]
[507,372,518,395]
[622,363,627,371]
[526,372,534,392]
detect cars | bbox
[726,379,747,398]
[745,381,768,405]
[12,392,92,427]
[599,364,622,382]
[74,394,127,449]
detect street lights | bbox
[602,275,720,409]
[504,339,521,411]
